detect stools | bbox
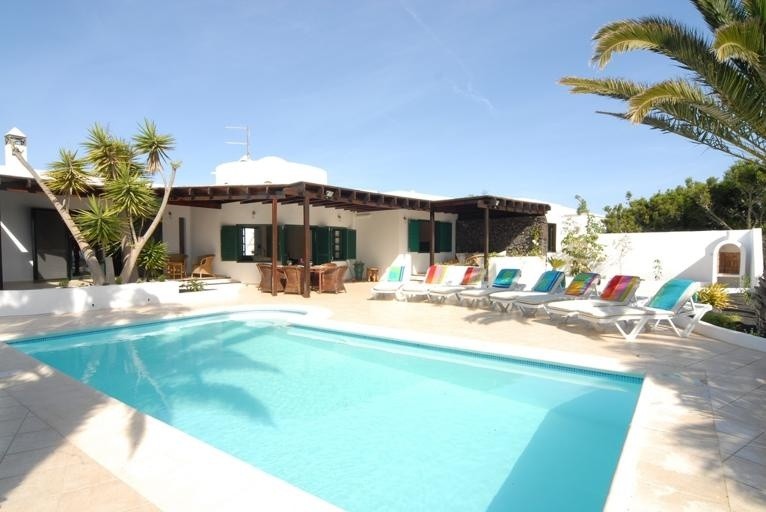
[367,266,379,282]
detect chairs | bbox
[373,253,566,314]
[191,254,215,279]
[578,279,713,341]
[547,276,641,331]
[166,253,187,279]
[257,261,349,294]
[516,271,600,319]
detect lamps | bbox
[494,199,500,209]
[27,179,38,193]
[324,189,334,199]
